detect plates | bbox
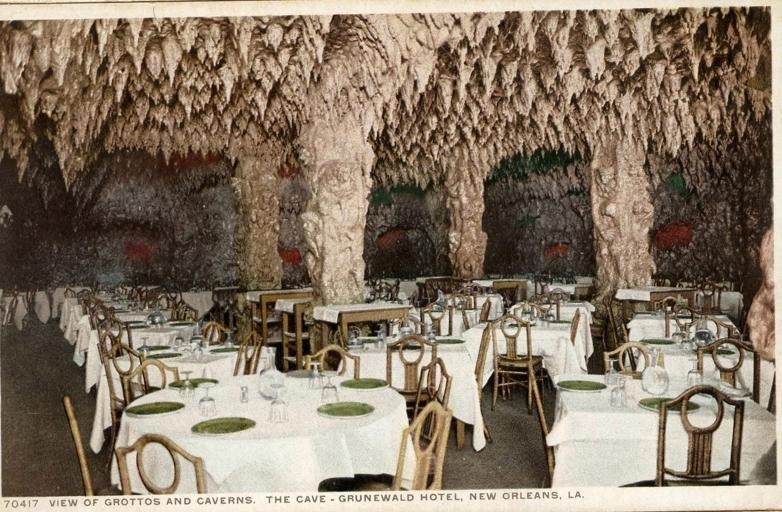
[556,379,607,392]
[188,417,255,434]
[638,397,700,415]
[339,377,388,391]
[728,392,752,400]
[123,401,185,417]
[315,401,375,418]
[640,337,675,347]
[167,377,219,390]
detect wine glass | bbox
[98,287,241,360]
[671,315,711,354]
[609,375,629,408]
[363,287,556,351]
[605,358,617,387]
[180,362,339,425]
[686,356,702,390]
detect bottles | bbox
[258,346,287,398]
